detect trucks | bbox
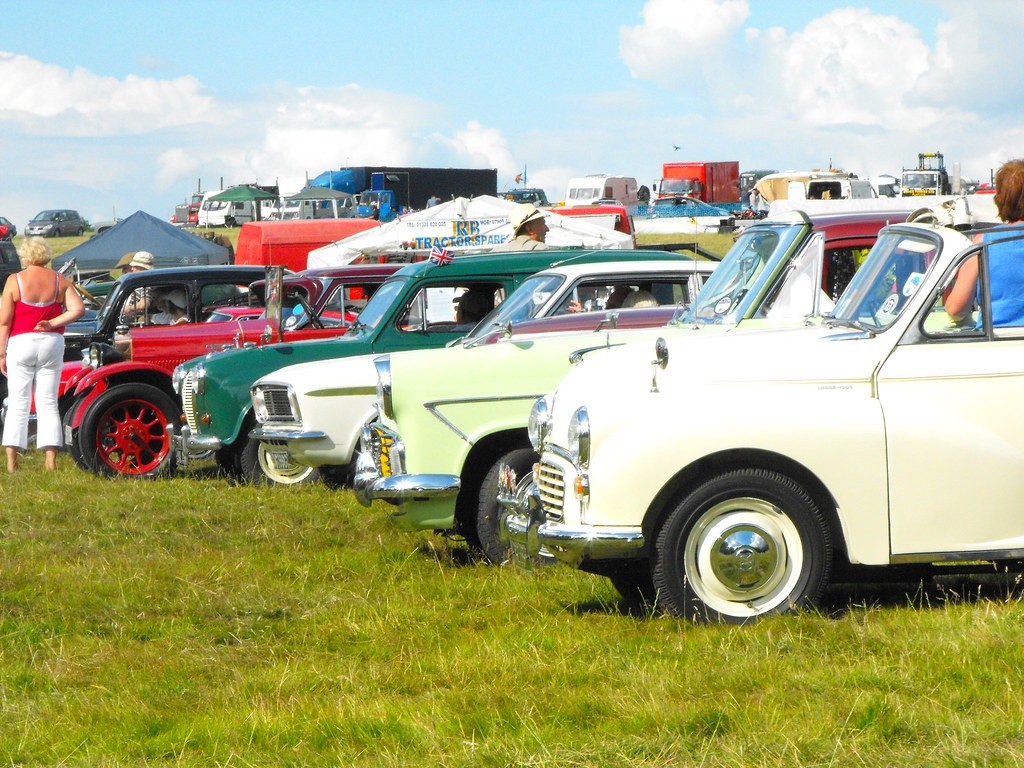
[653,161,739,202]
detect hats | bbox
[162,289,188,309]
[454,291,489,316]
[115,253,134,269]
[508,203,550,240]
[129,251,154,270]
[148,287,163,297]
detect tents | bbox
[205,186,357,230]
[51,210,229,276]
[306,194,633,271]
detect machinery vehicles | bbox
[900,153,951,197]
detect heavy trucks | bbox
[298,166,498,213]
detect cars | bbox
[529,219,1023,623]
[0,171,1024,564]
[0,217,18,241]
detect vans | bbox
[24,208,84,238]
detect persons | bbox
[115,252,154,322]
[449,287,494,331]
[489,202,551,253]
[809,195,816,200]
[426,193,441,209]
[0,236,85,474]
[742,188,760,210]
[138,287,191,325]
[569,287,660,313]
[942,160,1024,327]
[364,205,380,221]
[682,188,694,206]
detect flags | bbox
[428,245,455,266]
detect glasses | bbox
[455,306,468,313]
